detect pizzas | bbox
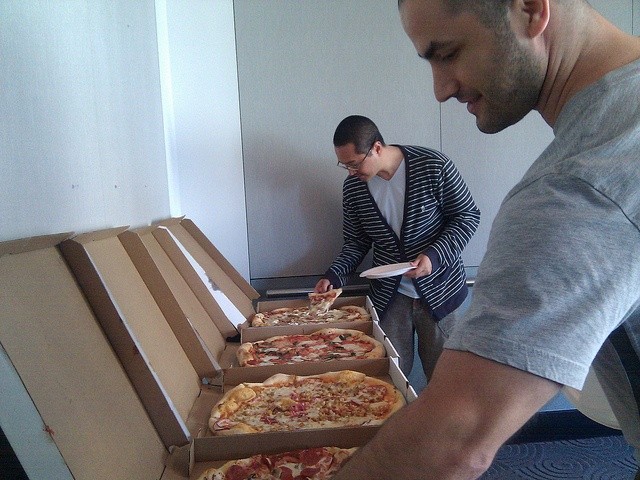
[251,303,373,328]
[206,369,405,436]
[230,327,388,367]
[191,447,359,480]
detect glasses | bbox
[337,140,383,170]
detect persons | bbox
[330,0,640,480]
[316,115,481,386]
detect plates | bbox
[359,262,421,280]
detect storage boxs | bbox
[130,225,399,366]
[1,233,382,480]
[155,215,380,325]
[73,224,418,439]
